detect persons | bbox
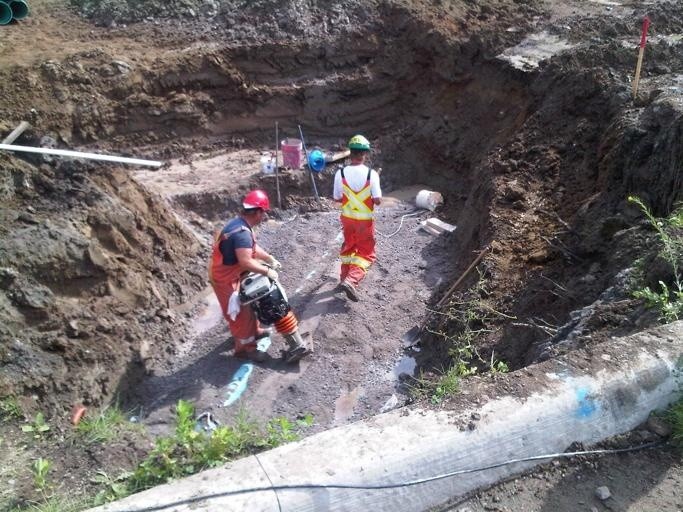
[208,189,281,363]
[333,134,382,302]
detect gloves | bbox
[267,268,278,280]
[266,254,282,268]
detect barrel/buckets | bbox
[281,138,302,170]
[414,190,444,212]
[260,152,275,174]
[8,0,29,21]
[309,147,325,171]
[0,0,14,26]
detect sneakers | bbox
[340,282,359,302]
[238,351,269,362]
[257,328,270,339]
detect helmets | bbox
[242,189,271,212]
[348,134,370,150]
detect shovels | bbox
[401,247,490,349]
